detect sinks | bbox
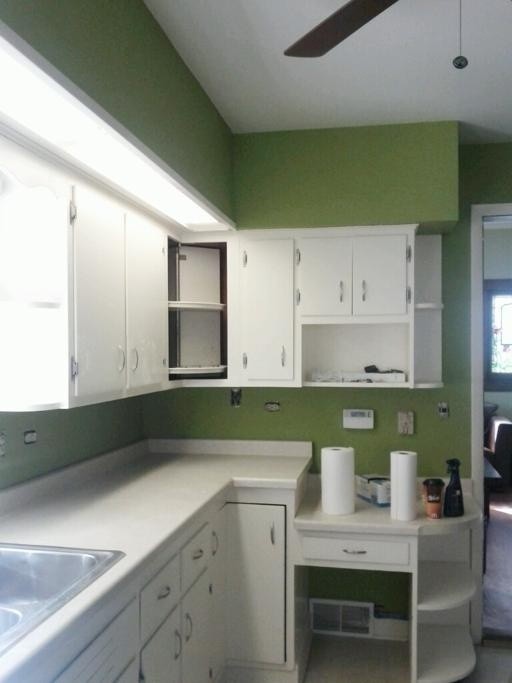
[0,542,125,607]
[0,605,21,656]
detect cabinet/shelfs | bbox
[167,222,232,390]
[233,229,303,388]
[0,180,169,411]
[226,487,315,681]
[140,504,227,680]
[300,227,413,323]
[414,230,445,390]
[294,527,477,682]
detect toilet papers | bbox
[390,450,420,521]
[320,446,355,515]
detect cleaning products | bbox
[442,458,463,518]
